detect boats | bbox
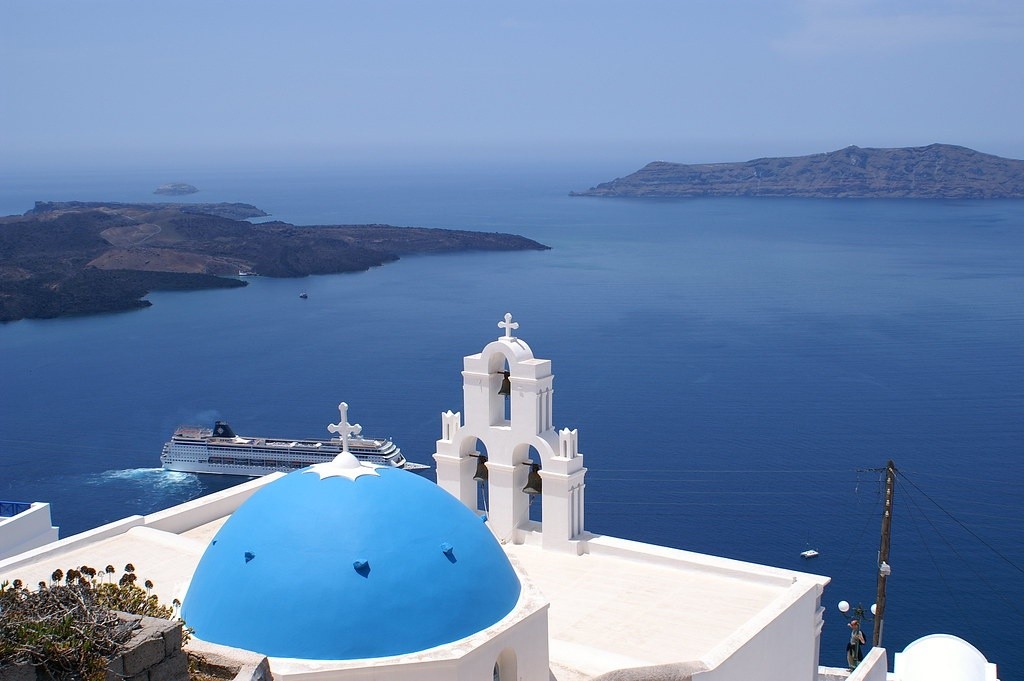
[160,421,431,476]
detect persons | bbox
[847,620,865,672]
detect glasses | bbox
[851,624,855,626]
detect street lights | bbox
[839,600,876,670]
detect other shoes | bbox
[846,670,853,672]
